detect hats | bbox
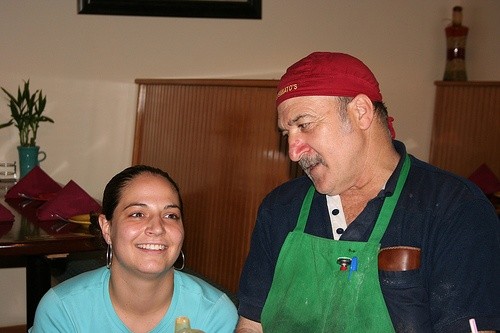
[275,52,396,136]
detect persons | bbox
[26,165,240,333]
[232,50,500,333]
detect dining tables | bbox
[0,226,109,333]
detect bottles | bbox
[443,7,468,82]
[0,163,23,241]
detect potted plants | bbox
[0,79,55,180]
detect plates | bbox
[68,214,91,228]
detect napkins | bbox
[0,167,104,225]
